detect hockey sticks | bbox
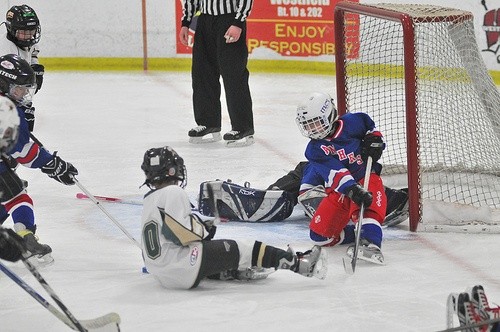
[435,318,500,332]
[29,132,142,249]
[76,193,231,223]
[342,156,373,275]
[0,253,121,332]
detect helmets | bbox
[4,3,42,49]
[0,53,36,93]
[142,147,182,185]
[296,93,333,123]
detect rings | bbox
[230,37,234,42]
[225,34,230,39]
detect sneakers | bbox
[188,123,221,143]
[224,128,255,147]
[347,242,384,266]
[16,230,52,257]
[449,285,500,332]
[289,243,330,280]
[231,265,274,280]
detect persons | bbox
[294,92,387,266]
[0,4,44,171]
[0,203,28,263]
[140,146,327,290]
[0,53,79,267]
[446,284,500,332]
[179,0,256,146]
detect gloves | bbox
[42,153,77,186]
[31,63,45,93]
[347,185,372,208]
[360,133,385,160]
[20,104,36,132]
